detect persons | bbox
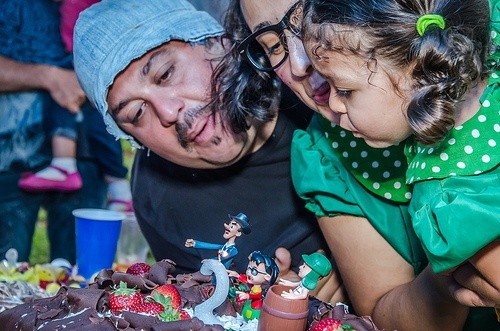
[18,0,102,192]
[292,0,500,305]
[199,0,500,331]
[73,0,346,306]
[0,0,107,265]
[185,213,332,309]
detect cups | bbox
[114,212,151,270]
[72,208,123,288]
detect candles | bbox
[194,259,229,325]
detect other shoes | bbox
[18,164,83,193]
[106,198,135,217]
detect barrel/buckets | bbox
[258,285,310,331]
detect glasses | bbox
[237,0,304,71]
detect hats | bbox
[73,1,228,156]
